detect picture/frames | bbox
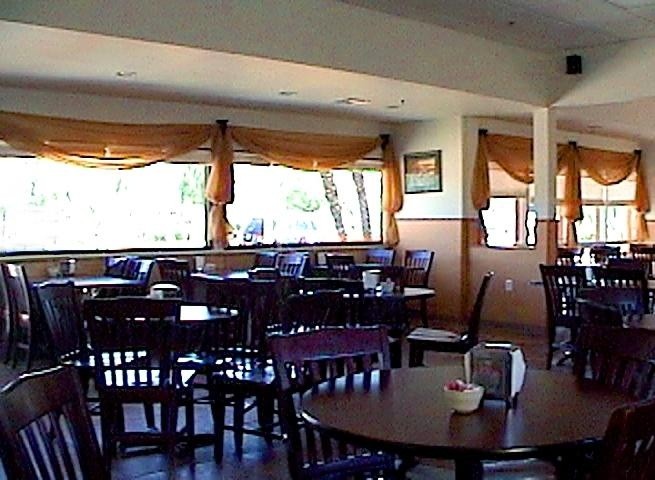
[403,150,442,194]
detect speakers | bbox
[567,56,582,74]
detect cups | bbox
[205,264,216,274]
[149,290,163,299]
[44,260,78,279]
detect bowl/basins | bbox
[448,410,485,440]
[442,380,485,415]
